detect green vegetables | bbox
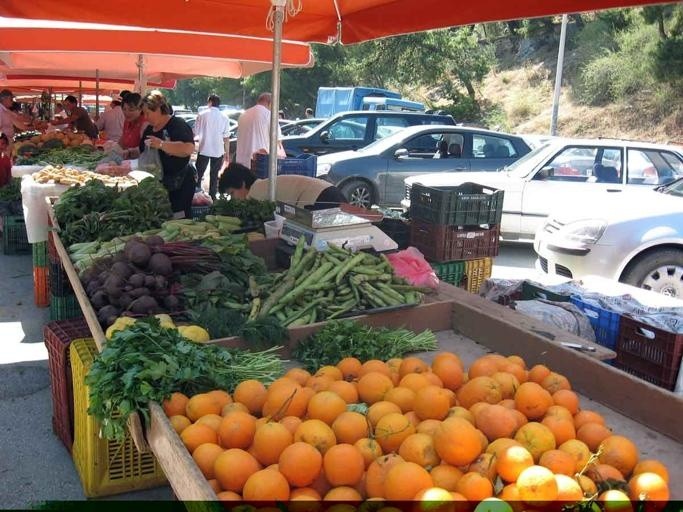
[46,174,175,246]
[294,319,439,374]
[79,315,291,443]
[158,237,274,314]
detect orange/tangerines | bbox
[162,352,670,511]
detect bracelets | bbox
[159,141,164,150]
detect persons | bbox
[218,162,350,211]
[95,95,125,142]
[195,94,231,199]
[235,92,282,169]
[139,89,196,219]
[0,132,11,187]
[0,88,31,138]
[117,92,149,160]
[50,95,99,141]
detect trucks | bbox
[314,85,425,117]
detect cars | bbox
[536,183,683,295]
[195,117,313,151]
[403,137,682,250]
[278,118,326,137]
[315,125,532,205]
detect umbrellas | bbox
[0,0,682,209]
[0,16,312,100]
[0,69,177,123]
[0,78,138,110]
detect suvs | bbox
[276,112,457,157]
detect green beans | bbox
[248,231,433,328]
